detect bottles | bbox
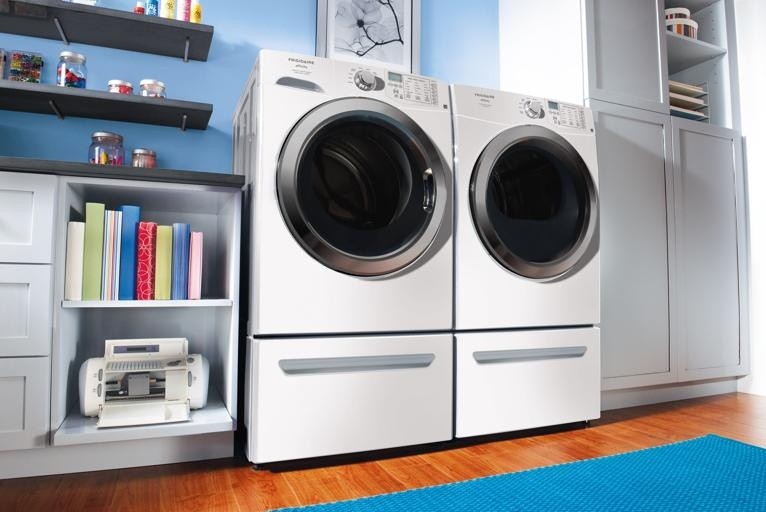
[133,1,202,24]
[88,131,124,166]
[55,51,88,89]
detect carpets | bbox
[262,429,765,512]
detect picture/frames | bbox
[313,0,424,78]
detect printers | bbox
[78,337,210,430]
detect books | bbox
[63,199,205,302]
[668,80,706,99]
[669,92,704,111]
[669,105,705,121]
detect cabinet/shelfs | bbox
[0,1,214,133]
[584,99,752,397]
[0,155,64,483]
[497,0,735,131]
[50,156,248,476]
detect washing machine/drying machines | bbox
[232,50,455,468]
[450,84,601,438]
